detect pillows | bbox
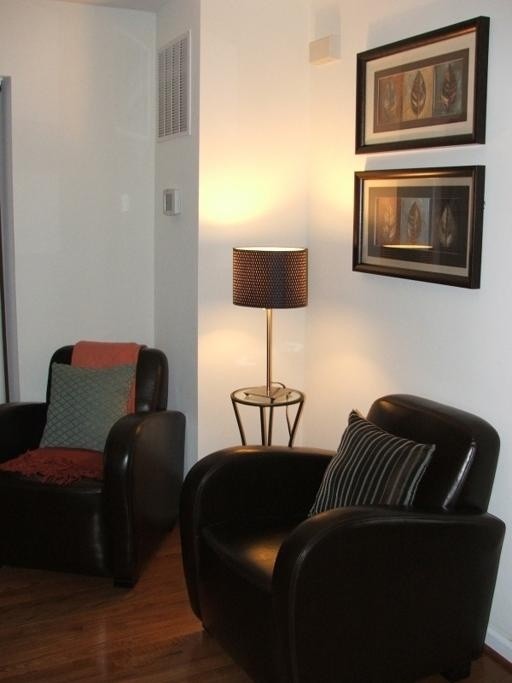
[306,410,437,519]
[39,363,134,452]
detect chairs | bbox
[0,345,187,585]
[180,394,506,683]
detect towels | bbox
[0,340,141,483]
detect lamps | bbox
[232,247,308,399]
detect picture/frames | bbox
[355,16,490,155]
[351,164,485,289]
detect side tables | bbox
[230,386,304,447]
